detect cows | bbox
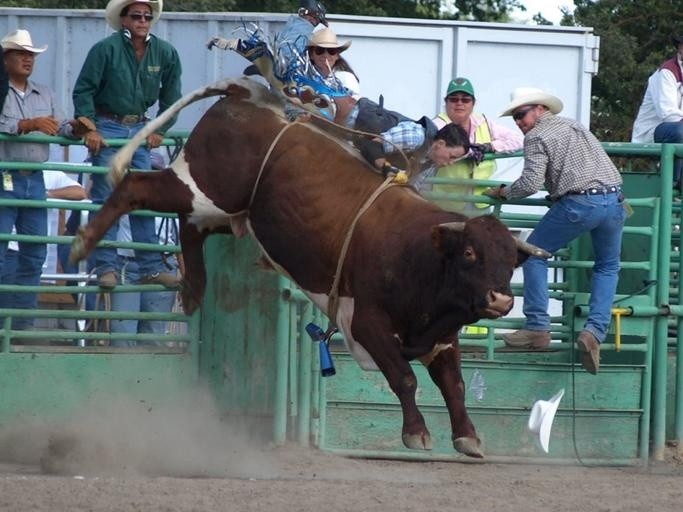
[65,73,555,462]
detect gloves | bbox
[381,160,409,186]
[480,181,509,204]
[463,141,496,166]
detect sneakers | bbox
[575,330,601,375]
[500,328,552,349]
[141,271,181,289]
[8,324,51,347]
[96,270,118,289]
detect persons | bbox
[108,152,185,345]
[483,84,628,377]
[0,40,10,118]
[8,168,87,280]
[431,77,525,333]
[274,1,329,59]
[292,26,359,97]
[630,34,683,205]
[72,0,181,290]
[56,155,107,348]
[209,32,471,193]
[0,28,97,347]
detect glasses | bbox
[313,46,338,56]
[125,13,154,21]
[513,105,537,121]
[447,97,472,104]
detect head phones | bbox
[124,29,151,43]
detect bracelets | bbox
[45,188,48,198]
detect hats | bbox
[104,0,164,32]
[446,78,475,98]
[670,34,683,49]
[499,87,564,118]
[299,0,329,27]
[0,29,49,55]
[303,26,352,54]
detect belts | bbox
[95,110,147,124]
[13,168,38,176]
[566,185,623,196]
